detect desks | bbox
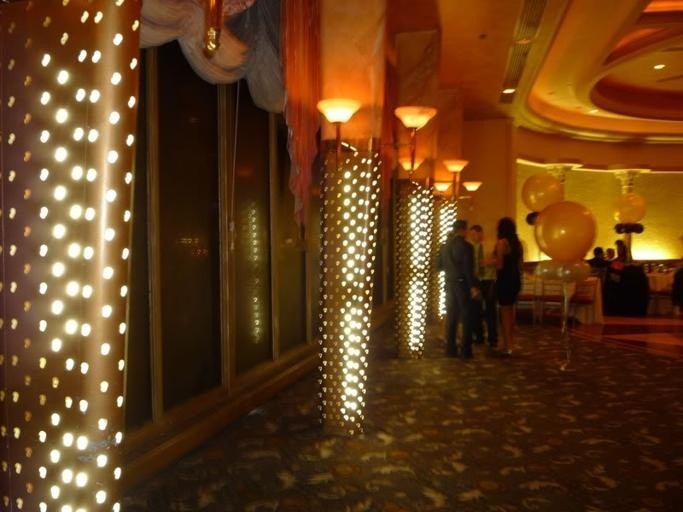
[647,272,675,316]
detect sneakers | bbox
[444,337,514,358]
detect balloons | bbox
[520,172,564,216]
[533,201,595,268]
[610,193,646,224]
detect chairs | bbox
[513,266,603,329]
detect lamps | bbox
[433,181,453,196]
[394,105,437,169]
[316,97,362,170]
[462,181,481,192]
[443,159,469,193]
[399,156,425,185]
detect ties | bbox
[476,242,485,278]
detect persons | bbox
[432,219,481,359]
[605,248,616,262]
[482,216,522,359]
[465,223,501,350]
[583,246,609,268]
